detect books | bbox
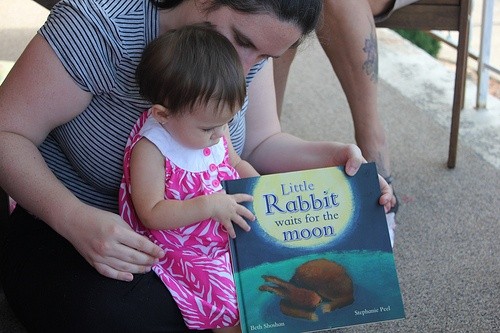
[221,162,406,332]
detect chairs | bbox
[376,0,472,169]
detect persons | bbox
[272,0,414,214]
[118,22,264,333]
[0,0,325,333]
